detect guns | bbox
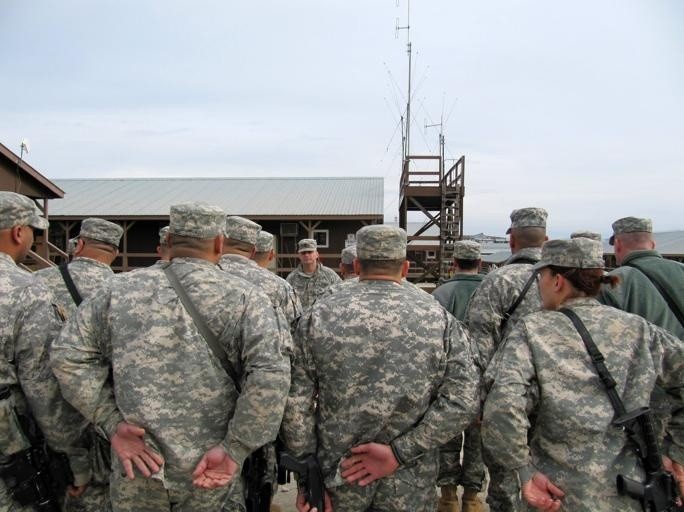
[246,451,273,511]
[0,447,58,512]
[277,451,324,511]
[613,407,675,512]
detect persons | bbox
[1,190,684,512]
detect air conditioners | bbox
[308,229,329,248]
[279,223,299,238]
[426,251,436,259]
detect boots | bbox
[461,486,484,512]
[438,486,460,512]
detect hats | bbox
[506,207,549,235]
[0,190,50,231]
[67,217,125,247]
[255,231,274,253]
[169,200,227,239]
[356,225,408,260]
[159,225,170,243]
[340,245,356,264]
[298,239,318,253]
[452,240,482,260]
[227,215,263,246]
[609,217,653,246]
[526,237,607,270]
